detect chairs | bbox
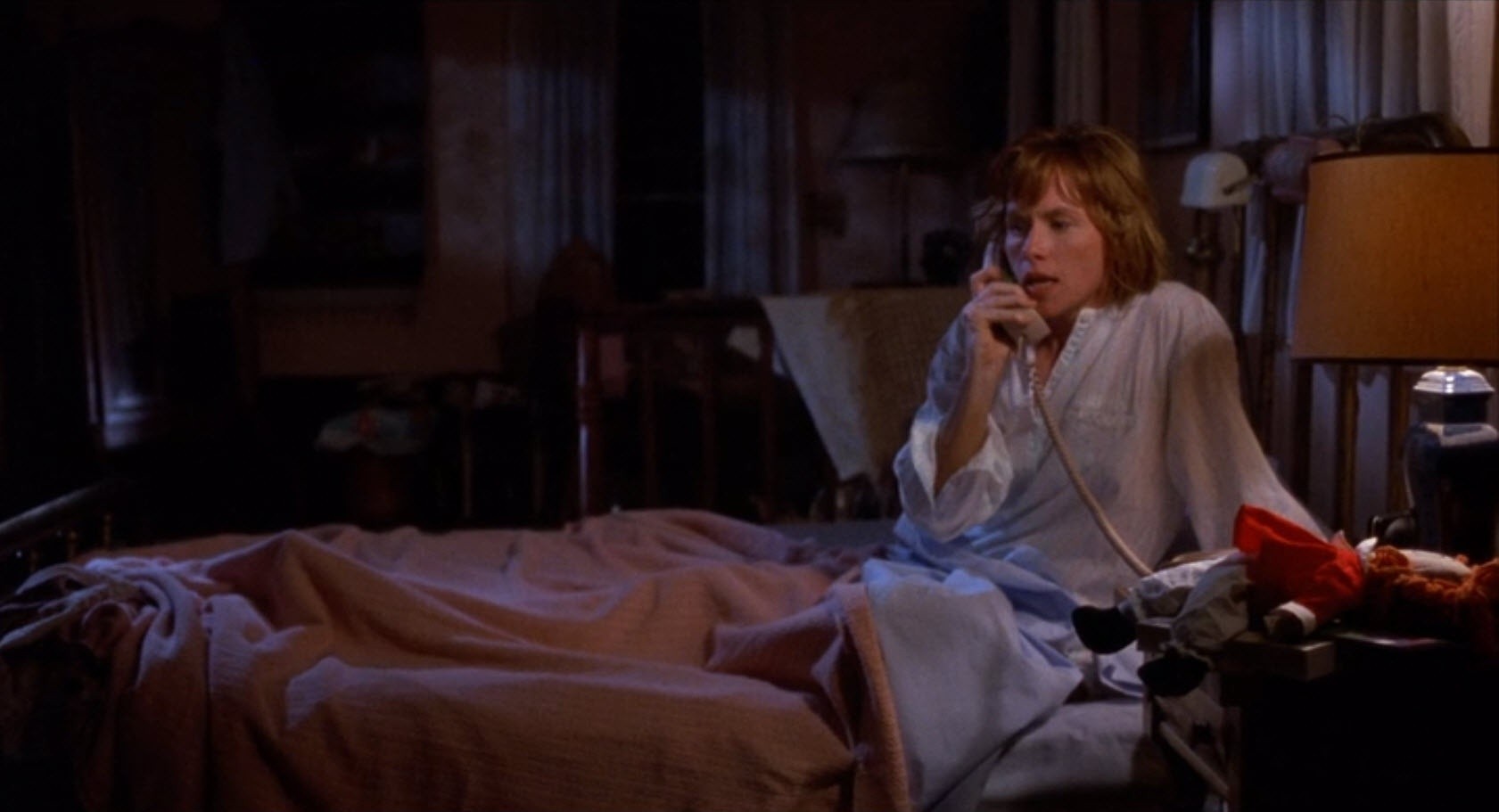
[552,297,776,527]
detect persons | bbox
[719,122,1324,812]
[1071,504,1499,697]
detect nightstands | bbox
[1148,654,1498,811]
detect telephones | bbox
[985,236,1049,346]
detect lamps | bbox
[1287,149,1499,548]
[831,83,955,288]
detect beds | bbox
[0,113,1472,812]
